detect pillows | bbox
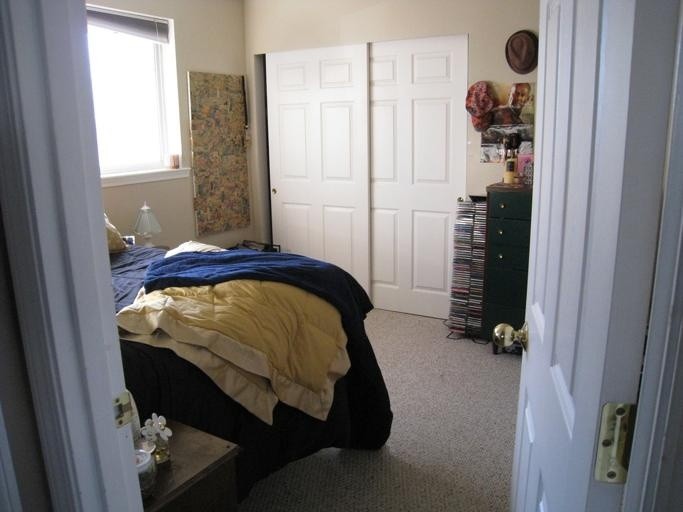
[104,213,128,252]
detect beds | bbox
[109,244,394,503]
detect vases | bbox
[155,441,170,464]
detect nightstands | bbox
[140,416,238,512]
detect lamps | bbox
[133,200,162,246]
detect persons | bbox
[507,82,534,125]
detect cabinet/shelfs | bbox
[481,181,532,355]
[253,35,468,322]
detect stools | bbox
[444,319,490,346]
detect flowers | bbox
[140,413,173,442]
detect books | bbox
[444,198,488,334]
